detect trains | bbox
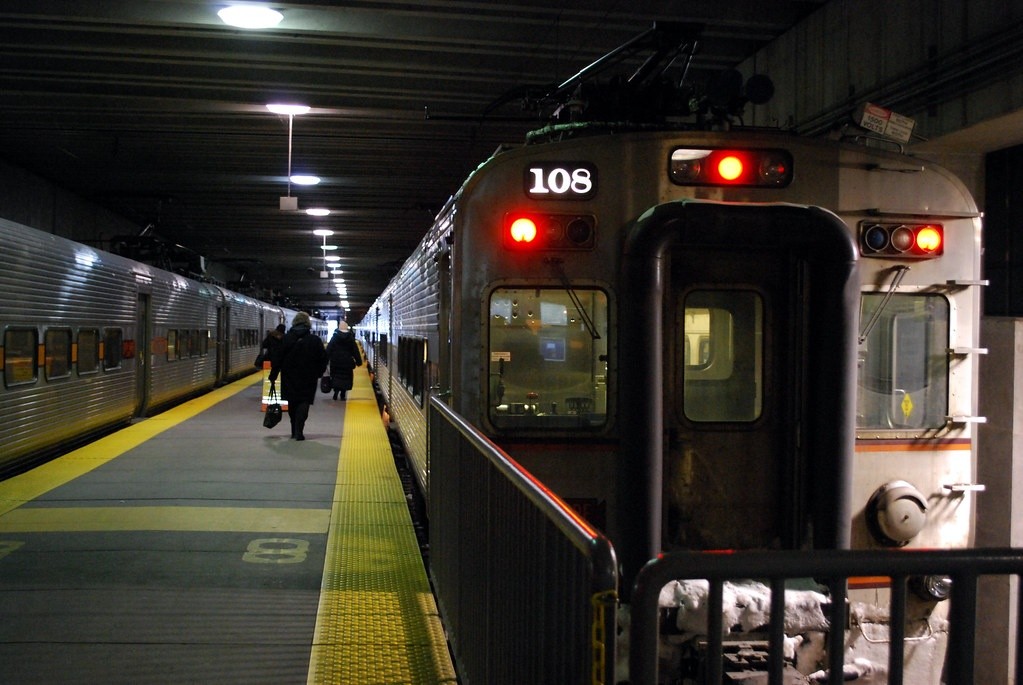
[0,219,328,483]
[355,22,989,685]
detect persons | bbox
[325,322,362,400]
[268,312,328,441]
[261,324,286,365]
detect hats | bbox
[339,321,349,333]
[275,324,286,334]
[291,312,311,327]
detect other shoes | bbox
[296,431,306,442]
[333,392,338,400]
[340,397,347,401]
[289,431,297,438]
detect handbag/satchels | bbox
[320,374,332,394]
[254,354,264,371]
[263,383,282,429]
